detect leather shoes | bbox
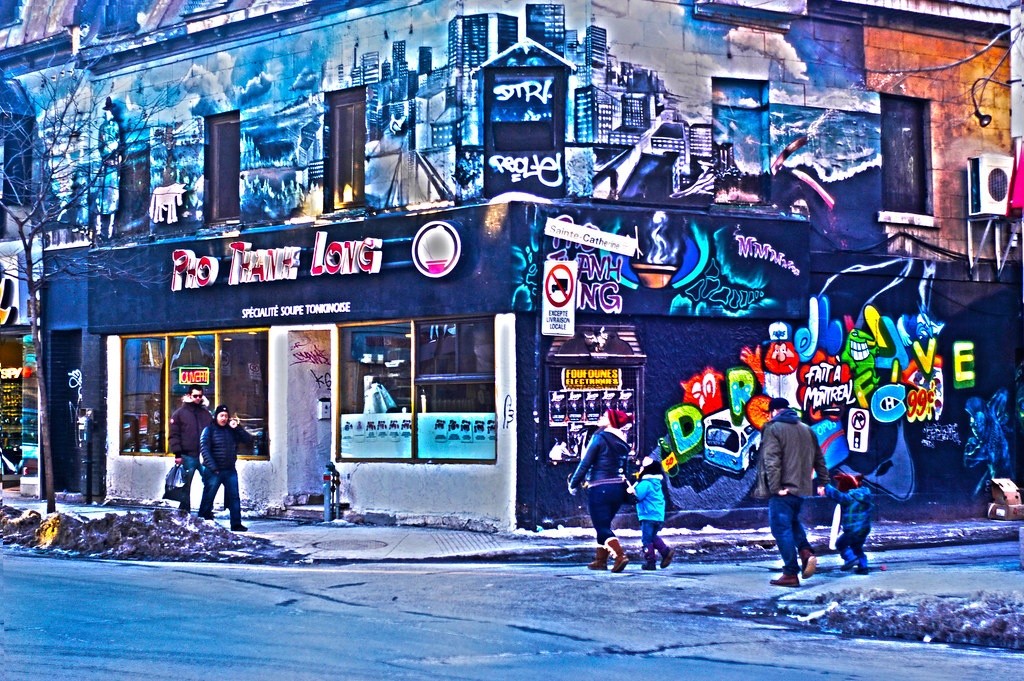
[770,574,799,586]
[799,549,817,579]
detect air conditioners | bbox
[968,155,1016,216]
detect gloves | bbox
[175,458,183,463]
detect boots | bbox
[642,543,656,569]
[588,544,610,570]
[654,538,674,568]
[603,537,629,572]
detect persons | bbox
[752,397,829,587]
[199,405,255,531]
[567,408,632,574]
[170,383,215,519]
[362,362,396,414]
[630,457,674,571]
[818,471,873,573]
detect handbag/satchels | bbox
[162,463,186,502]
[166,463,186,487]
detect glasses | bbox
[191,395,202,398]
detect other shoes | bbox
[231,523,248,531]
[854,568,868,574]
[841,558,859,571]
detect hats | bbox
[834,473,858,491]
[606,408,628,428]
[215,405,230,419]
[638,456,662,482]
[769,398,789,410]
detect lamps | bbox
[972,77,1011,127]
